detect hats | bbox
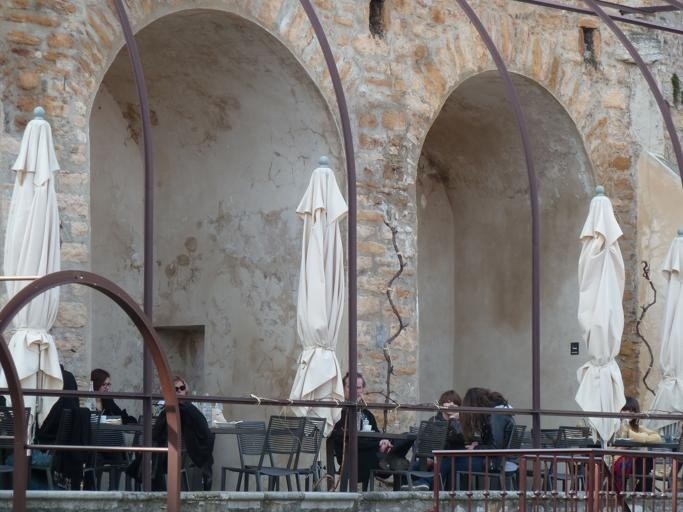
[90,368,110,391]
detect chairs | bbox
[1,406,204,491]
[220,416,682,491]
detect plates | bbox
[215,420,242,429]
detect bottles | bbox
[85,380,97,422]
[202,392,212,429]
[362,417,369,425]
[191,391,201,413]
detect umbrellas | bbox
[575,185,628,448]
[643,228,683,441]
[3,107,65,455]
[288,156,348,461]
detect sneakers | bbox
[400,480,431,490]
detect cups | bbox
[97,415,107,423]
[213,402,223,415]
[362,424,372,432]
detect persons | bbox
[152,375,215,490]
[91,368,137,424]
[604,396,661,512]
[379,386,516,491]
[332,371,394,482]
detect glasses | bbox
[175,386,186,392]
[102,382,111,387]
